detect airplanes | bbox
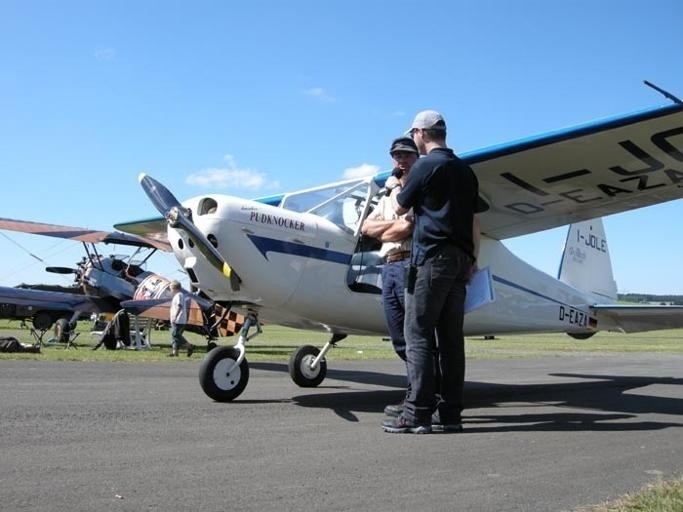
[111,79,682,399]
[1,216,248,355]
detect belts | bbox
[384,249,409,265]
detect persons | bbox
[361,110,481,435]
[166,280,196,357]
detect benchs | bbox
[91,308,154,351]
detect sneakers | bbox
[379,403,463,433]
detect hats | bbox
[404,110,447,135]
[390,138,420,159]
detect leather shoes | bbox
[187,343,196,357]
[167,354,178,357]
